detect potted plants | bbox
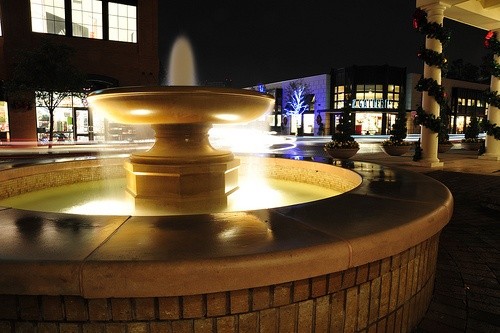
[460,105,483,151]
[380,108,414,157]
[325,107,360,159]
[438,123,454,154]
